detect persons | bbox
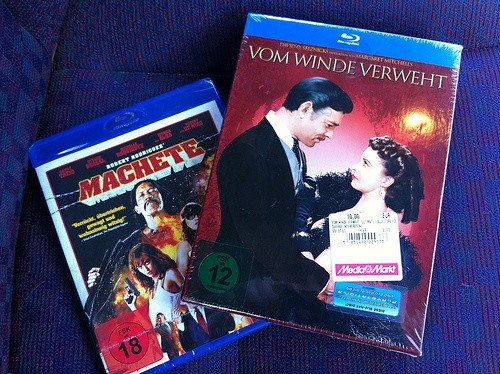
[216,77,424,303]
[89,180,253,361]
